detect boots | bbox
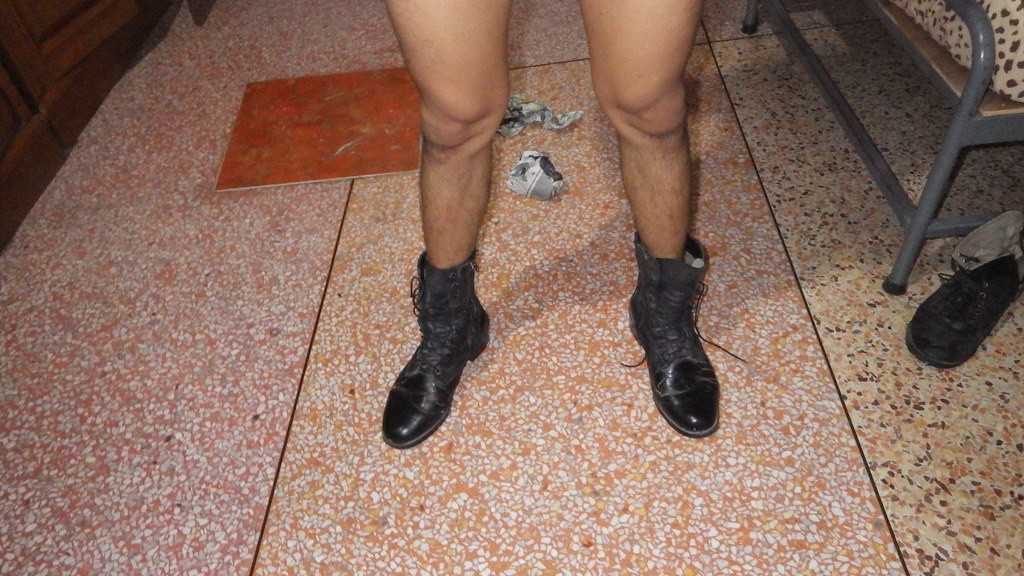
[908,210,1023,366]
[381,249,490,448]
[620,232,746,437]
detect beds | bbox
[743,0,1023,293]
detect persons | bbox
[381,0,747,448]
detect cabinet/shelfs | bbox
[0,0,213,252]
